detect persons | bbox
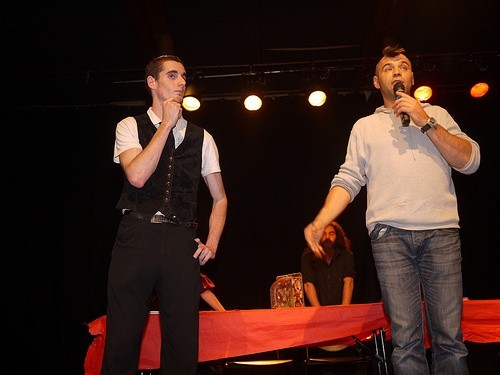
[200,273,226,310]
[304,46,480,375]
[301,222,355,307]
[101,55,228,375]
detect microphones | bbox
[393,82,411,126]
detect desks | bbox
[83,300,500,375]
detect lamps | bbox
[414,86,433,101]
[308,82,327,107]
[182,84,201,111]
[242,87,262,111]
[470,82,489,98]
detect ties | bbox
[159,122,176,150]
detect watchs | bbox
[421,117,437,134]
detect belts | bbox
[124,210,196,226]
